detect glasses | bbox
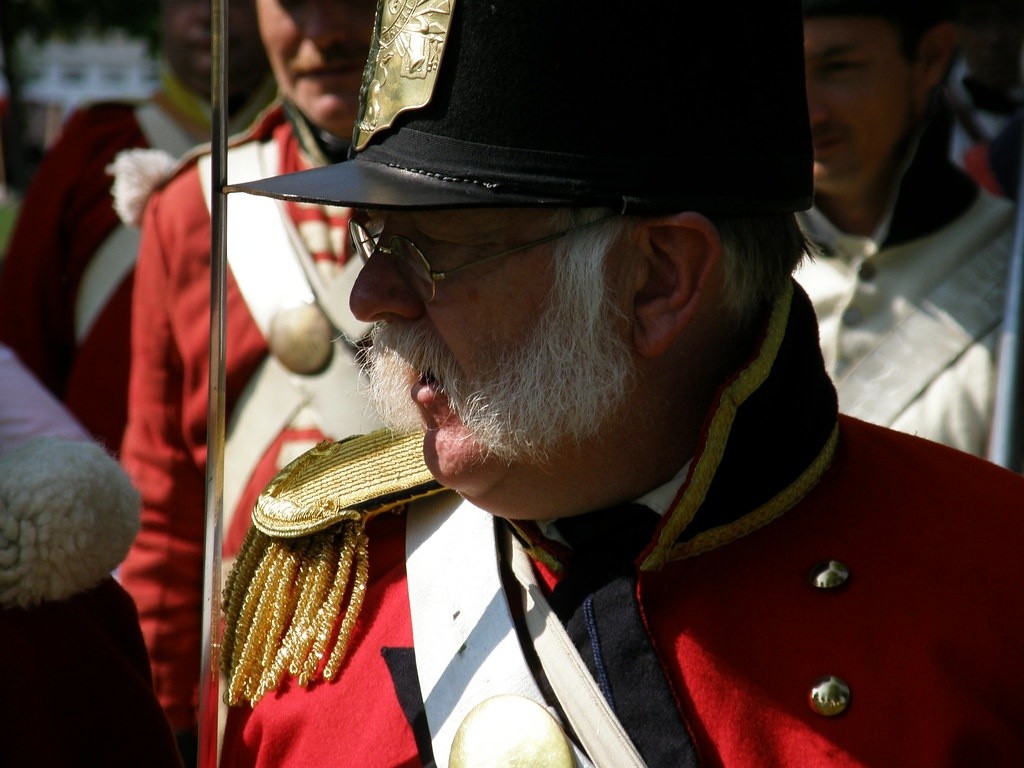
[349,212,624,304]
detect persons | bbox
[0,0,1024,768]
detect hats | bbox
[223,0,816,211]
